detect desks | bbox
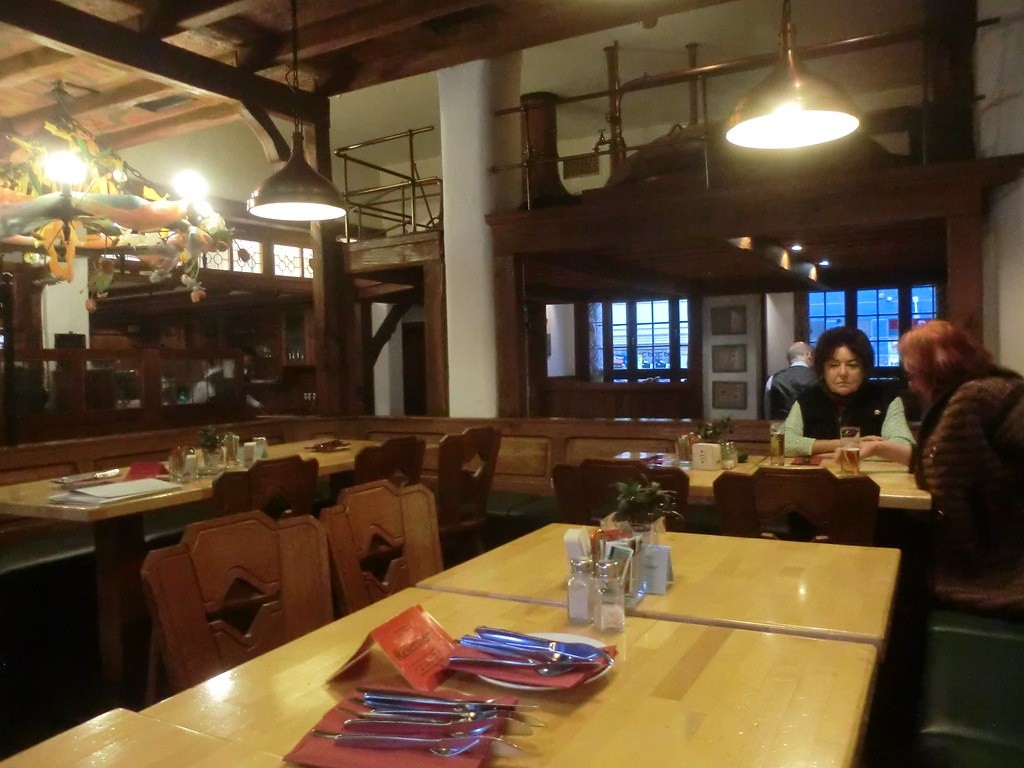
[0,697,304,768]
[415,524,901,661]
[0,437,440,693]
[613,451,765,505]
[139,588,877,768]
[746,456,932,510]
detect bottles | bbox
[722,442,738,469]
[170,446,196,481]
[244,437,266,468]
[567,556,595,625]
[595,560,624,631]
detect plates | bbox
[477,633,615,692]
[316,440,350,451]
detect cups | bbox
[840,426,861,474]
[225,435,240,470]
[771,423,785,467]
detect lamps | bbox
[799,260,819,281]
[0,77,229,313]
[724,0,860,150]
[763,244,792,270]
[247,0,348,222]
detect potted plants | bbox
[608,473,683,544]
[697,418,734,442]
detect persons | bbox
[764,342,822,421]
[189,358,232,405]
[772,326,916,463]
[838,321,1024,613]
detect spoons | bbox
[313,686,546,757]
[450,626,614,676]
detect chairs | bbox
[929,546,1024,617]
[353,437,427,486]
[321,480,442,614]
[914,609,1024,768]
[213,453,320,519]
[552,459,689,534]
[439,426,503,574]
[139,511,335,691]
[712,467,881,547]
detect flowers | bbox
[677,432,700,446]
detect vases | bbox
[674,444,693,467]
